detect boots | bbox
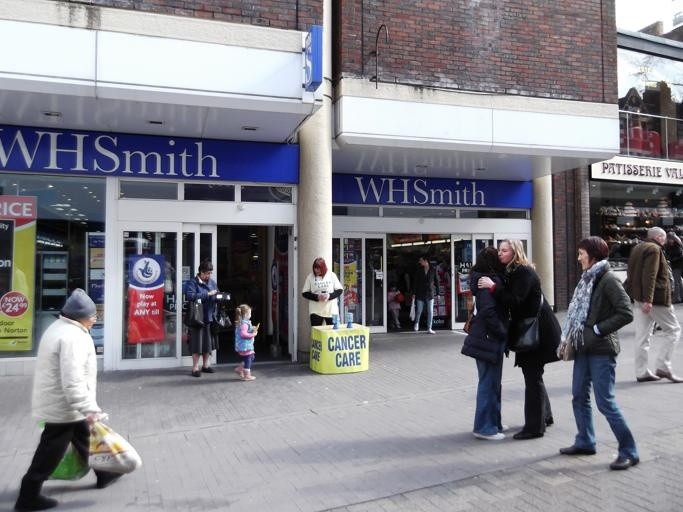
[94,469,122,488]
[240,368,256,381]
[15,479,58,511]
[234,362,244,378]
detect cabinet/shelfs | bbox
[595,199,683,261]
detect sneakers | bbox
[471,428,506,441]
[414,322,419,331]
[427,328,435,334]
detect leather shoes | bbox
[560,444,596,455]
[544,417,554,426]
[192,368,200,377]
[656,367,683,383]
[636,372,660,382]
[201,366,216,373]
[513,429,544,440]
[610,453,640,470]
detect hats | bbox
[61,288,98,320]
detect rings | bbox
[645,307,648,309]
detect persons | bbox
[184,261,223,376]
[624,226,681,383]
[14,287,125,509]
[460,247,508,440]
[478,236,563,438]
[664,230,681,303]
[412,254,440,334]
[387,284,404,330]
[302,257,342,326]
[234,304,258,380]
[555,236,639,469]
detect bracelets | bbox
[436,295,440,297]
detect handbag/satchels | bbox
[210,291,235,335]
[39,420,90,480]
[556,336,576,362]
[184,292,206,329]
[508,318,540,352]
[463,313,476,334]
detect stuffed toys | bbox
[395,293,403,303]
